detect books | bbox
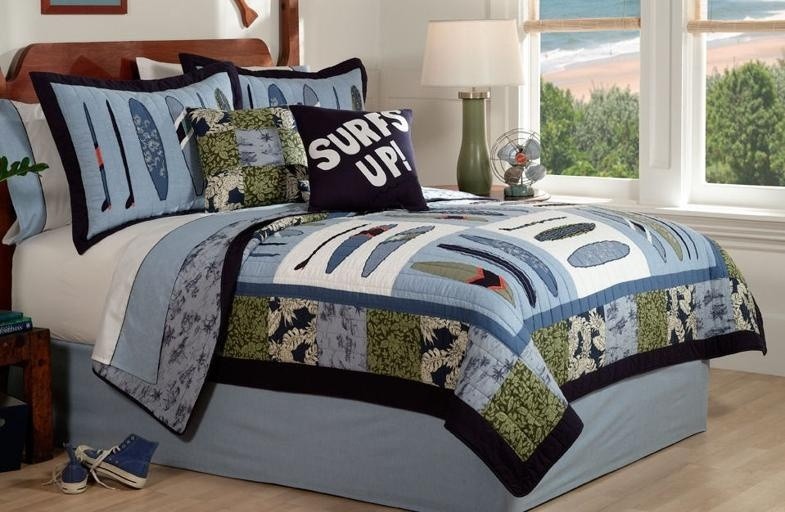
[0,310,34,336]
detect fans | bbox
[488,127,547,201]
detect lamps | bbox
[421,17,525,196]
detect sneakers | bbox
[75,433,159,490]
[42,442,90,494]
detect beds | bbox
[0,39,711,511]
